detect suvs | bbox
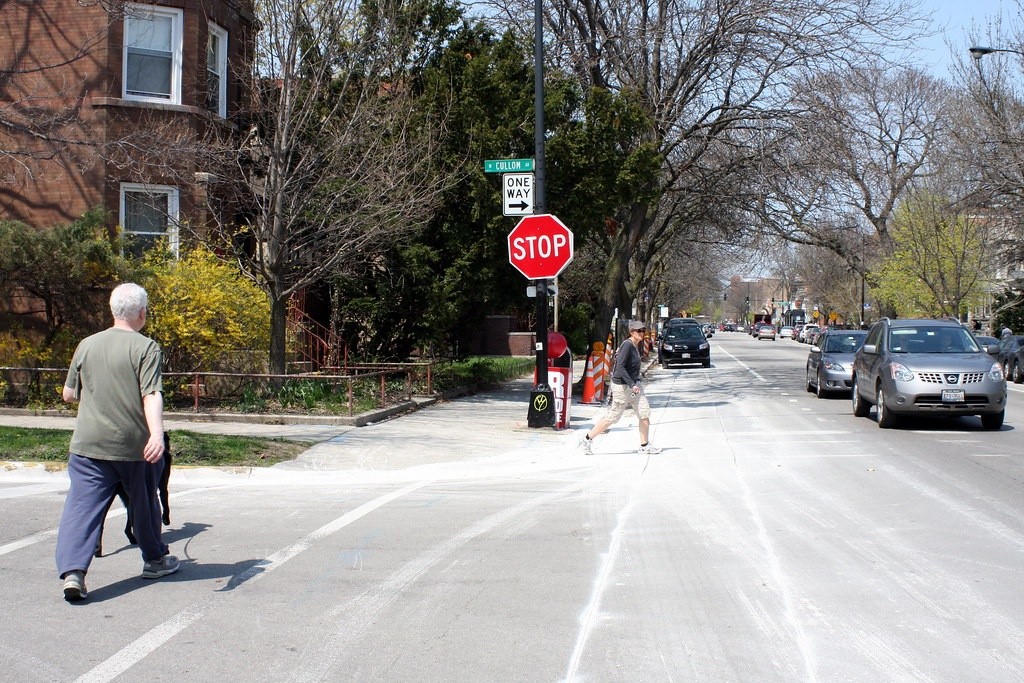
[852,317,1008,430]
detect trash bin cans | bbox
[534,332,573,428]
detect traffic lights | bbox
[746,296,750,304]
[771,297,775,305]
[724,293,727,301]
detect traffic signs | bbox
[503,171,535,217]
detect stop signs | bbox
[507,213,574,280]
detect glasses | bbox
[634,329,645,333]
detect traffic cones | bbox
[578,356,601,406]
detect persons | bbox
[579,322,663,455]
[1002,325,1013,339]
[900,336,909,351]
[938,333,953,351]
[58,283,184,606]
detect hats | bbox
[629,321,646,331]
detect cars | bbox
[805,330,869,399]
[758,326,776,341]
[749,321,776,338]
[975,334,1024,384]
[657,323,713,369]
[780,326,794,339]
[719,323,734,332]
[665,317,705,338]
[795,324,841,346]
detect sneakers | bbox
[143,555,180,578]
[578,436,592,454]
[640,442,662,454]
[62,571,87,599]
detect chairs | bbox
[671,331,682,338]
[925,335,943,351]
